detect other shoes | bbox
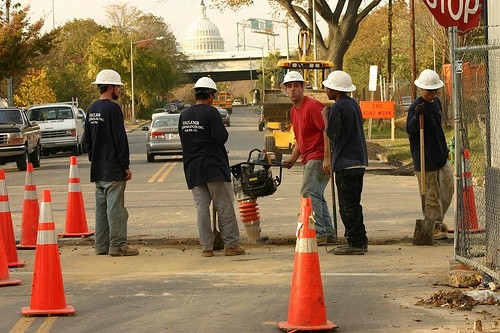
[334,244,364,254]
[202,249,213,257]
[327,239,336,243]
[225,246,245,256]
[434,228,447,239]
[111,245,139,256]
[316,237,327,245]
[362,249,368,252]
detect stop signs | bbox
[421,1,482,35]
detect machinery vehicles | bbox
[262,58,335,166]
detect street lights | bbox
[132,37,164,121]
[247,17,291,58]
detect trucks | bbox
[212,91,233,113]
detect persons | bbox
[406,68,455,240]
[321,70,368,255]
[83,69,139,256]
[178,77,245,257]
[280,71,335,247]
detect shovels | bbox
[413,113,434,246]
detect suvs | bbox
[232,99,242,107]
[0,102,87,171]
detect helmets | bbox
[191,76,221,94]
[91,69,125,86]
[280,71,307,89]
[322,71,356,92]
[414,69,444,90]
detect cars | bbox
[258,110,266,132]
[151,102,192,120]
[397,97,416,112]
[141,115,182,162]
[214,107,230,127]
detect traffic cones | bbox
[57,157,94,238]
[275,195,339,333]
[17,162,40,250]
[448,148,487,234]
[0,168,26,287]
[21,189,77,317]
